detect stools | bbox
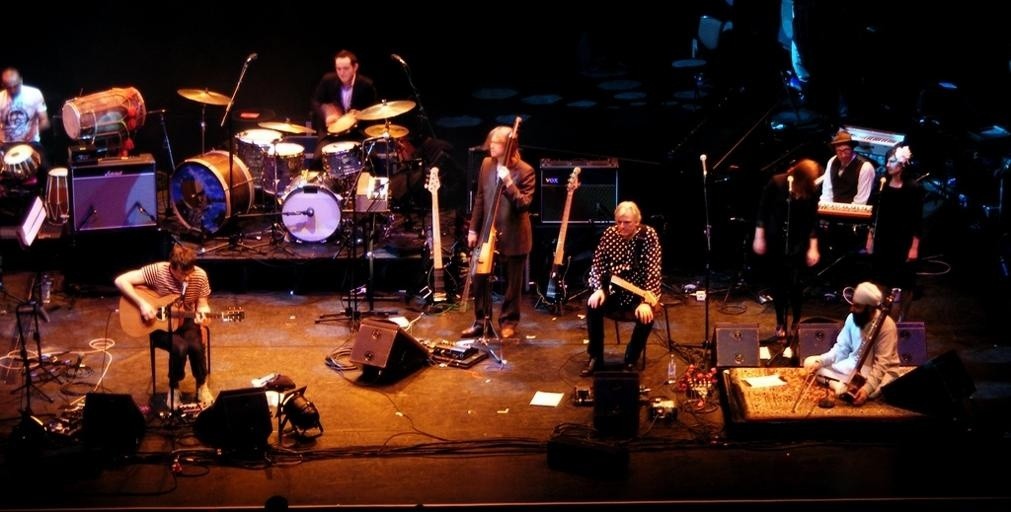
[149,324,209,393]
[606,308,647,371]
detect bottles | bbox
[41,275,52,304]
[667,353,677,384]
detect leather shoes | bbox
[501,329,514,338]
[625,356,636,370]
[580,358,605,376]
[462,319,485,336]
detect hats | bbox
[830,131,859,148]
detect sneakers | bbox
[198,387,213,402]
[167,388,181,410]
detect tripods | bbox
[0,299,63,433]
[195,140,297,256]
[315,184,400,324]
[148,295,195,450]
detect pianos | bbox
[817,199,874,224]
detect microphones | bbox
[366,131,391,141]
[391,54,407,66]
[700,154,708,175]
[787,175,794,191]
[181,280,189,302]
[247,53,257,62]
[879,177,887,191]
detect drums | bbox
[233,127,282,188]
[1,143,42,180]
[44,166,70,227]
[62,87,146,140]
[263,143,303,195]
[323,141,366,179]
[280,168,342,243]
[172,149,253,233]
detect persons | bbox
[874,143,926,300]
[461,124,537,340]
[113,241,218,410]
[1,67,50,193]
[820,130,877,207]
[304,50,381,192]
[577,200,665,378]
[751,158,825,343]
[804,280,901,406]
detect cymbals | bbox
[258,121,315,135]
[364,124,408,139]
[357,100,416,120]
[177,88,233,105]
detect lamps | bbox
[75,372,323,470]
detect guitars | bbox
[418,166,463,315]
[540,164,583,310]
[602,270,660,307]
[119,285,244,339]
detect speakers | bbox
[896,321,927,366]
[540,158,618,224]
[594,362,640,439]
[190,387,273,448]
[81,392,146,460]
[69,154,157,235]
[711,325,760,367]
[798,322,841,367]
[348,319,430,376]
